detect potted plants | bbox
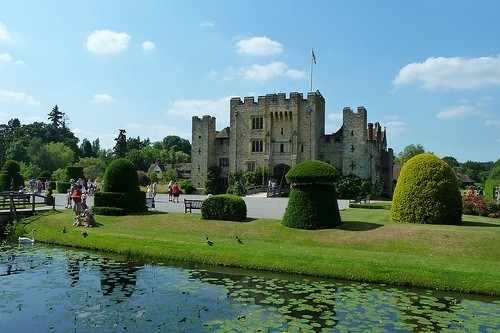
[43,186,54,206]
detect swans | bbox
[18,229,37,243]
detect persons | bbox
[268,177,278,193]
[478,186,482,196]
[495,187,500,199]
[147,179,181,203]
[469,187,474,196]
[19,176,103,228]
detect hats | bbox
[69,179,74,183]
[88,179,91,182]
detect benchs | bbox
[361,195,371,204]
[184,198,203,213]
[350,196,362,205]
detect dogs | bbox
[73,209,95,227]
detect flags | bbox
[312,50,316,64]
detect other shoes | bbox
[177,201,179,203]
[169,199,172,202]
[172,201,175,203]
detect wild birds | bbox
[83,231,88,238]
[62,225,67,233]
[205,236,213,246]
[235,235,242,244]
